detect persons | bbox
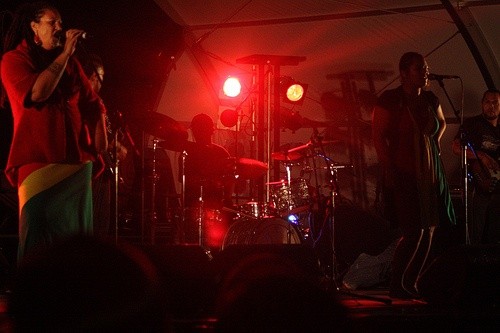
[4,230,167,333]
[0,0,128,265]
[452,89,500,243]
[177,113,233,204]
[372,51,455,300]
[219,277,351,333]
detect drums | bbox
[238,202,277,216]
[266,177,312,216]
[181,206,229,248]
[222,216,305,251]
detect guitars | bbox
[469,157,500,193]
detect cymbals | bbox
[288,140,337,152]
[157,138,207,156]
[141,110,189,140]
[271,151,301,161]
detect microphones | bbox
[427,73,461,79]
[56,29,95,39]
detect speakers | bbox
[145,243,322,314]
[416,238,500,315]
